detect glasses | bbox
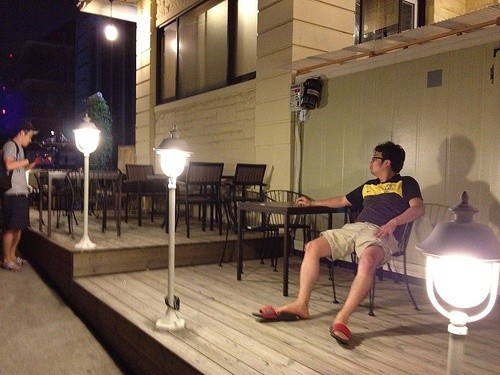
[371,156,383,160]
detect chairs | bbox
[331,205,420,317]
[125,163,155,180]
[218,189,315,272]
[175,162,268,238]
[66,165,123,237]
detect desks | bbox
[236,201,346,298]
[39,171,114,237]
[124,179,165,227]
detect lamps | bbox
[72,114,102,157]
[104,0,118,41]
[300,78,323,110]
[415,191,500,327]
[153,125,195,182]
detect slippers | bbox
[252,305,296,321]
[329,322,353,344]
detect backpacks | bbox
[0,140,19,193]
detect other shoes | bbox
[0,260,21,272]
[11,256,27,266]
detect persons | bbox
[0,121,42,273]
[252,142,426,344]
